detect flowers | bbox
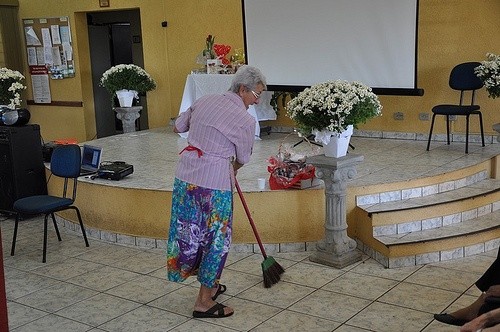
[0,66,28,110]
[475,52,500,99]
[97,62,158,110]
[201,34,215,59]
[282,79,383,135]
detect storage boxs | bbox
[206,59,223,74]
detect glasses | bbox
[245,84,262,99]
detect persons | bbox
[434,245,500,332]
[166,65,268,318]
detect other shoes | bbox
[432,313,471,326]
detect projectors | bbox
[97,162,133,180]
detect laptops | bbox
[79,144,103,175]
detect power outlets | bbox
[393,112,403,120]
[419,112,430,120]
[448,114,458,121]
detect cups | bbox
[258,179,265,190]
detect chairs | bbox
[10,143,89,262]
[427,61,486,155]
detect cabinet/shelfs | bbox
[0,123,48,221]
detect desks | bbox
[176,72,277,141]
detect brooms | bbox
[233,175,287,289]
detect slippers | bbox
[193,301,235,318]
[211,283,227,302]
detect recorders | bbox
[0,106,31,127]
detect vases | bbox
[312,125,354,157]
[114,89,134,108]
